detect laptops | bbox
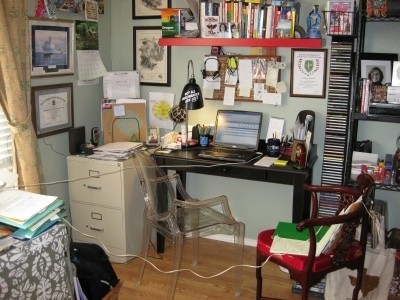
[197,109,263,162]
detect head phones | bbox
[202,58,221,80]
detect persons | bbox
[230,24,237,39]
[368,67,389,104]
[220,24,228,37]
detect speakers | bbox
[68,126,85,155]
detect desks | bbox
[150,144,318,254]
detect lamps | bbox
[175,58,204,150]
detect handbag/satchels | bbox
[69,243,118,300]
[324,215,395,300]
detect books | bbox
[322,157,345,187]
[327,0,355,36]
[356,78,370,114]
[254,156,280,168]
[331,41,352,75]
[239,1,298,38]
[326,114,348,135]
[324,135,347,157]
[319,191,342,218]
[328,88,350,115]
[329,75,351,88]
[220,1,240,23]
[201,1,220,16]
[160,9,180,38]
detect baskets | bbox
[322,8,355,38]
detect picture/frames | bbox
[32,25,69,70]
[131,0,172,20]
[289,49,328,99]
[359,58,391,102]
[132,26,171,87]
[30,82,74,138]
[392,61,400,103]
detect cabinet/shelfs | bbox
[67,158,125,262]
[345,0,400,194]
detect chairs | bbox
[254,184,370,300]
[128,150,246,300]
